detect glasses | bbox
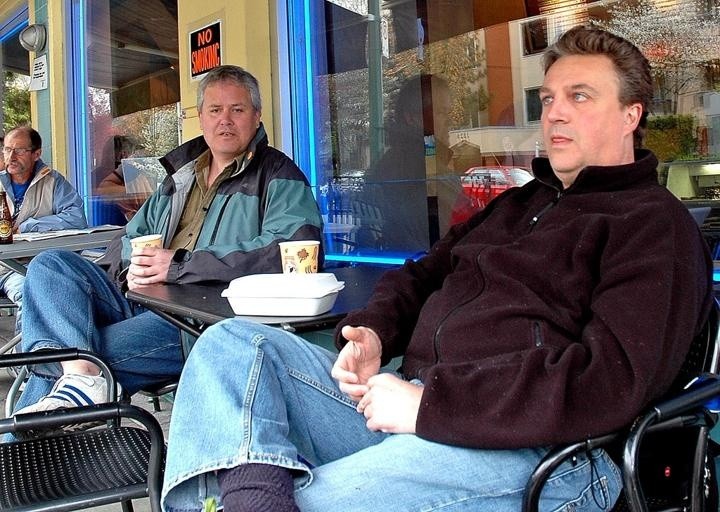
[2,147,35,154]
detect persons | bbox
[362,72,476,252]
[90,108,181,221]
[0,126,87,391]
[160,24,713,512]
[0,65,324,443]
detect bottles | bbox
[0,191,13,245]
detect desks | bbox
[124,265,404,340]
[0,226,127,418]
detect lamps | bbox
[16,22,44,53]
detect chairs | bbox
[0,350,168,512]
[524,293,719,511]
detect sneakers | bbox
[6,350,31,391]
[10,375,130,441]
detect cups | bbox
[278,241,321,274]
[130,234,164,251]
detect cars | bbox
[318,169,366,212]
[461,166,534,207]
